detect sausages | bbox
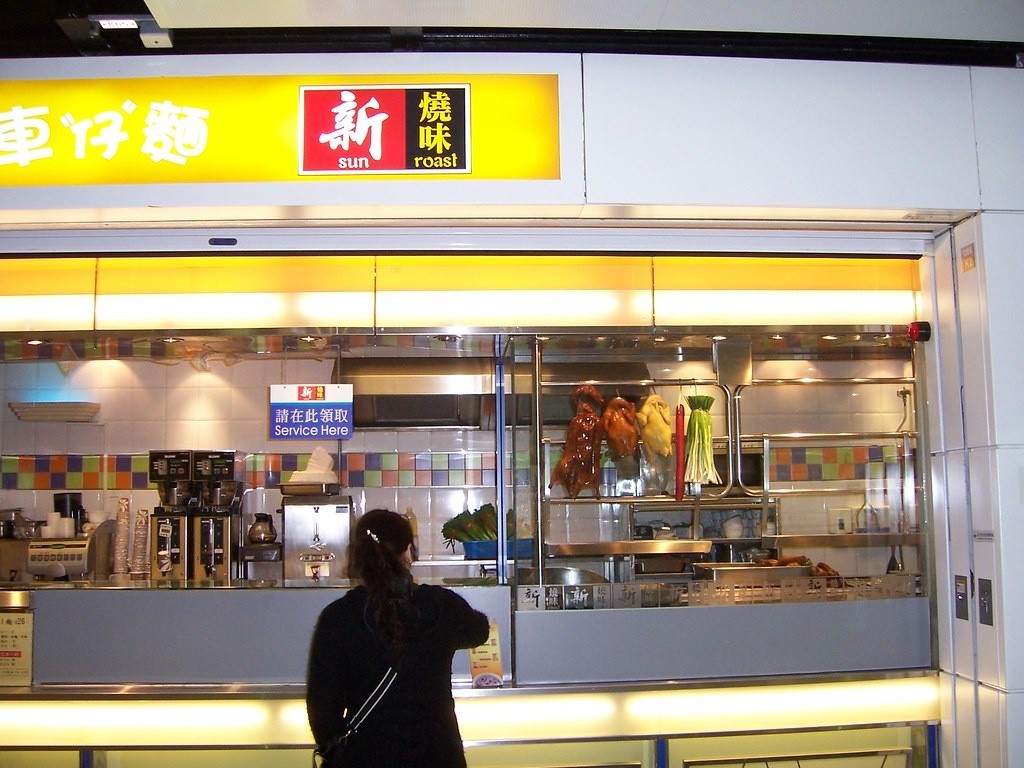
[675,404,685,502]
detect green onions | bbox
[440,503,516,554]
[683,395,722,484]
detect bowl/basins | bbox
[0,509,21,520]
[711,507,776,538]
[0,520,12,538]
[87,510,110,523]
[276,482,344,496]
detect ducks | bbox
[548,385,604,500]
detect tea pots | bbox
[248,511,277,543]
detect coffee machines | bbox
[190,449,246,580]
[148,449,192,583]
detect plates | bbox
[40,511,75,539]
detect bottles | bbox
[404,507,419,559]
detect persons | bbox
[306,509,490,768]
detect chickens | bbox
[635,395,672,461]
[602,396,641,461]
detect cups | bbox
[112,497,149,579]
[53,493,81,533]
